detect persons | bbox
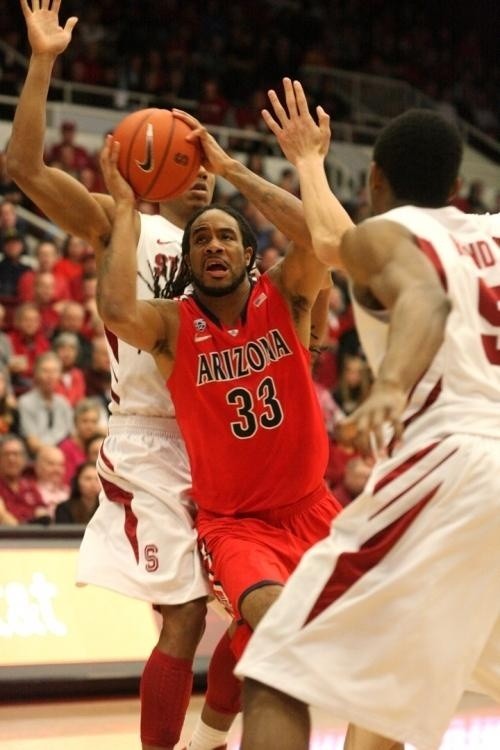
[95,106,402,749]
[262,75,363,271]
[0,125,111,525]
[2,1,499,155]
[464,181,491,213]
[313,275,377,509]
[231,152,301,270]
[230,104,499,750]
[0,0,331,749]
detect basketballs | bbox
[113,106,200,200]
